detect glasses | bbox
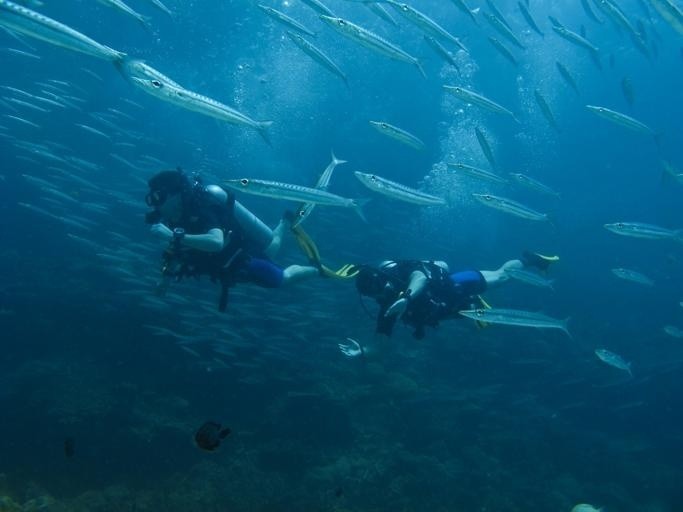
[145,190,173,207]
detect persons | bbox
[357,248,560,338]
[142,169,359,313]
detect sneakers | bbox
[284,210,296,226]
[310,258,330,278]
[531,255,549,270]
[472,295,483,310]
